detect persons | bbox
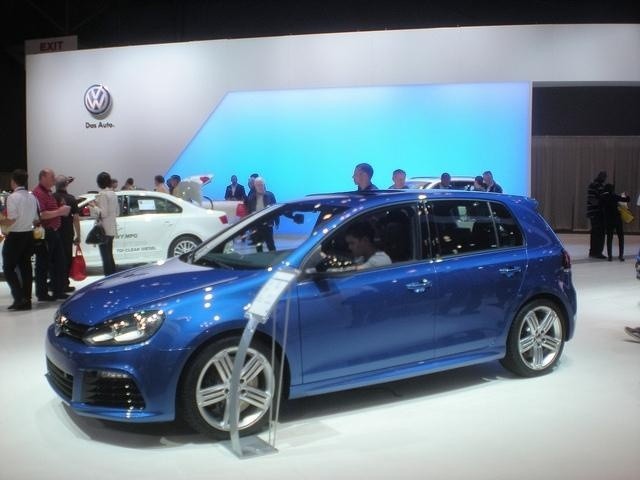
[32,169,73,301]
[111,173,279,254]
[634,193,640,279]
[434,172,453,189]
[603,182,631,261]
[386,169,411,192]
[482,171,504,193]
[86,172,122,277]
[0,169,41,311]
[352,162,379,194]
[585,169,610,259]
[340,223,393,272]
[473,175,488,193]
[431,253,567,320]
[46,174,82,292]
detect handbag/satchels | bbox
[85,224,106,244]
[69,256,86,280]
[33,225,51,256]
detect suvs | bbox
[45,190,576,441]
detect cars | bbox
[1,190,228,278]
[407,177,475,190]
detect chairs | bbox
[383,218,414,261]
